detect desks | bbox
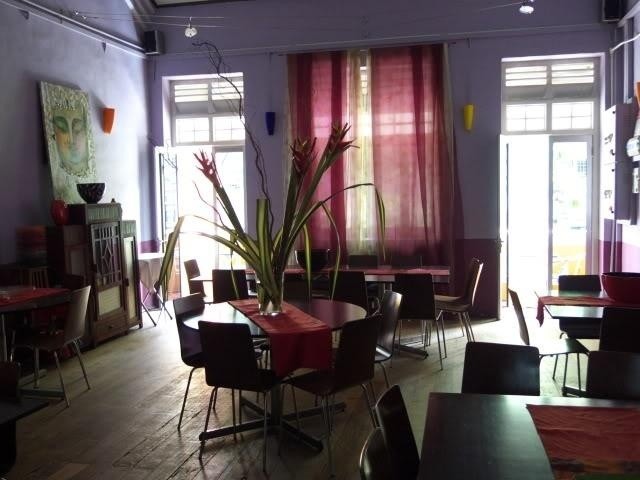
[0,287,70,414]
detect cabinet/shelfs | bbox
[49,204,143,345]
[601,103,636,226]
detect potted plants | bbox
[192,124,385,320]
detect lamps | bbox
[264,112,276,136]
[184,19,197,38]
[519,0,535,15]
[102,107,115,134]
[463,103,473,132]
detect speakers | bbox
[143,29,165,57]
[601,1,620,21]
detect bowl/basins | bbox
[76,183,105,204]
[295,249,330,271]
[602,272,640,300]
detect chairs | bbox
[10,284,93,407]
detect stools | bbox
[17,265,48,288]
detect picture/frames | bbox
[39,81,95,204]
